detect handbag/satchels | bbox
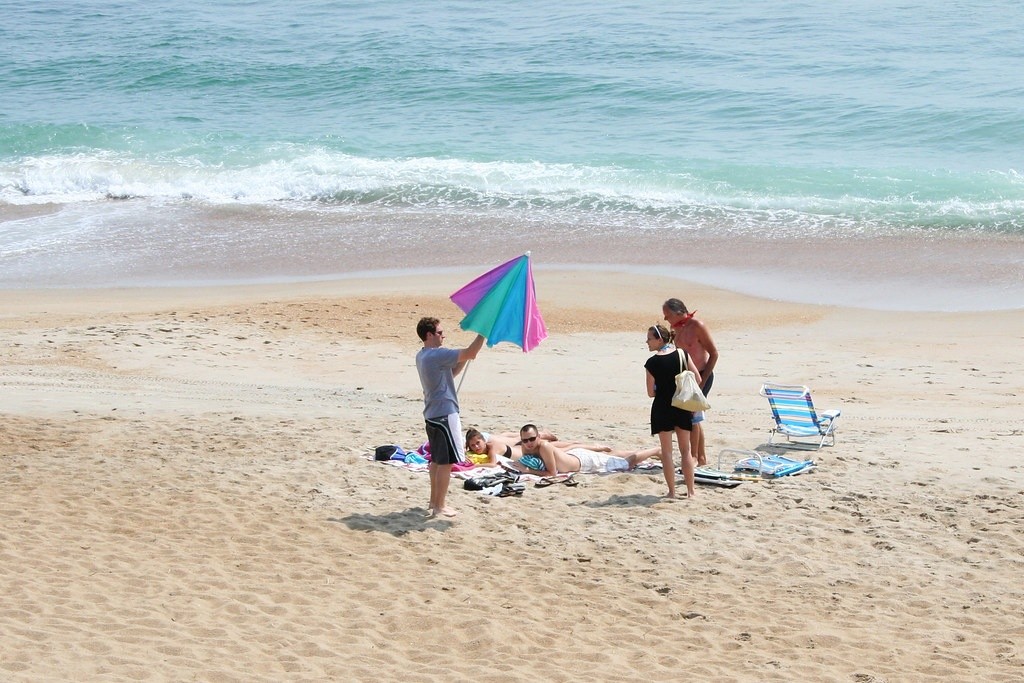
[671,348,712,412]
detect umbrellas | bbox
[450,250,549,400]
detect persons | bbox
[512,425,662,475]
[460,427,612,471]
[662,298,719,467]
[644,324,702,498]
[414,317,485,517]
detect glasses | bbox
[434,331,442,335]
[521,437,537,443]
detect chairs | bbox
[758,382,840,452]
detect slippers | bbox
[534,477,556,487]
[562,476,579,486]
[497,483,525,497]
